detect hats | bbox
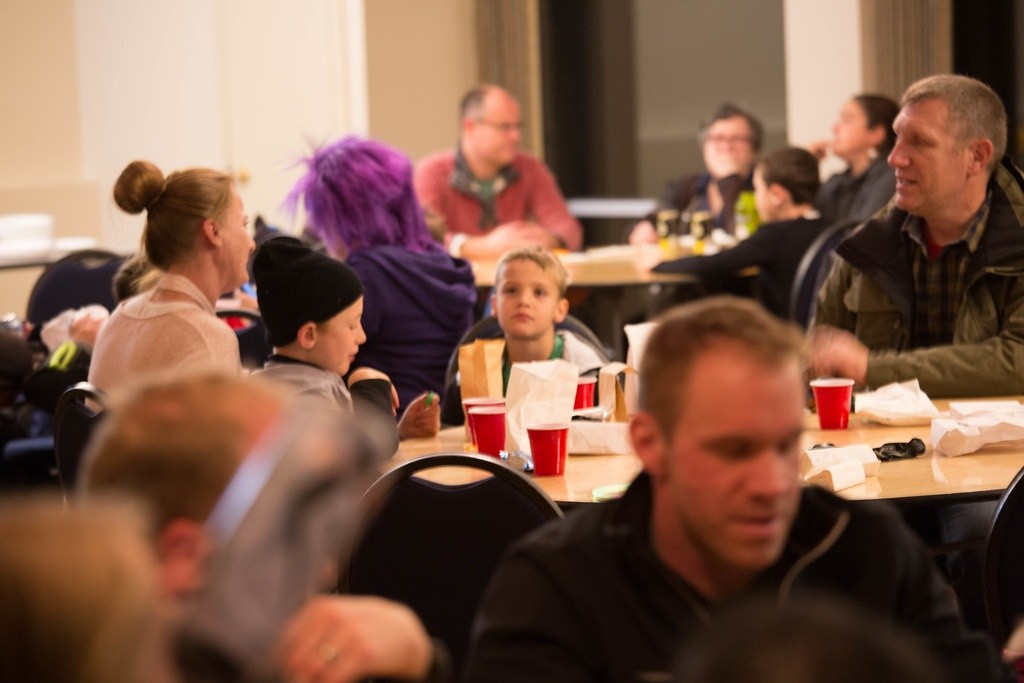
[251,236,362,346]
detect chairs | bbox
[441,310,609,395]
[338,451,565,668]
[789,219,866,327]
[54,381,108,496]
[215,310,267,372]
[980,468,1024,650]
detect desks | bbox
[376,397,1023,633]
[469,245,760,317]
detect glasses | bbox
[475,117,524,138]
[697,135,753,154]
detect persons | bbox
[0,74,1024,683]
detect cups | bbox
[809,377,856,430]
[573,376,597,410]
[526,423,570,478]
[462,398,506,459]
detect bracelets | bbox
[450,233,467,259]
